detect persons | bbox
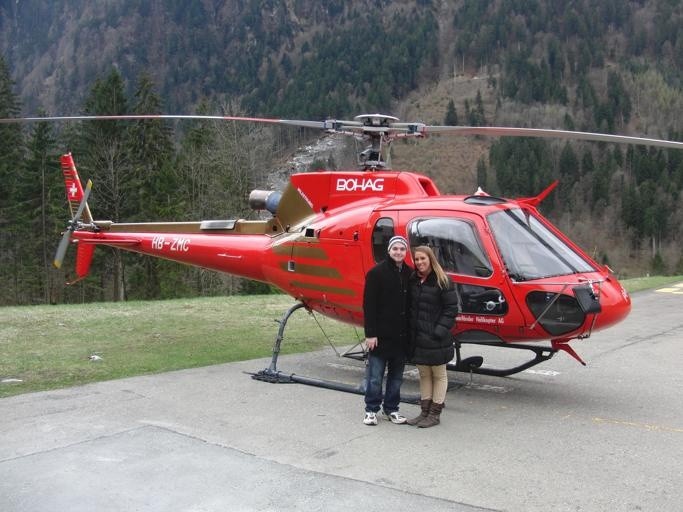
[408,246,457,428]
[362,235,416,424]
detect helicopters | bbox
[0,113,683,410]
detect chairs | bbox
[449,227,504,303]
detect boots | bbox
[416,401,445,428]
[407,398,433,426]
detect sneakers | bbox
[381,406,407,425]
[362,411,378,425]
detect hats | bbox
[387,236,408,253]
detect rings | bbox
[368,344,371,346]
